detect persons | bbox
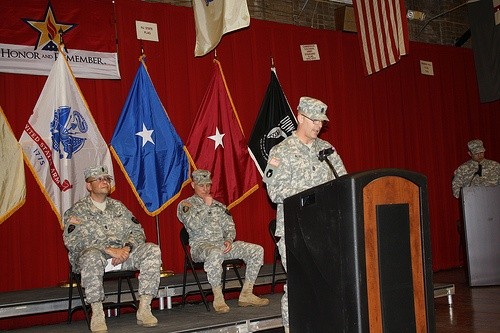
[177,170,269,313]
[452,140,500,198]
[63,167,162,332]
[262,96,348,333]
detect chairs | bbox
[269,220,287,293]
[68,266,141,330]
[180,227,245,312]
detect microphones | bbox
[318,147,336,161]
[478,164,482,176]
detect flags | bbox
[352,0,409,78]
[247,63,299,179]
[18,47,115,232]
[0,108,26,223]
[183,59,259,213]
[191,0,250,57]
[110,61,192,216]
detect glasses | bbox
[300,112,328,126]
[88,177,112,182]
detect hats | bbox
[296,96,330,121]
[466,139,486,155]
[84,165,113,181]
[191,168,213,186]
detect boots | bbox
[136,295,159,327]
[89,301,108,332]
[212,286,230,313]
[238,282,269,306]
